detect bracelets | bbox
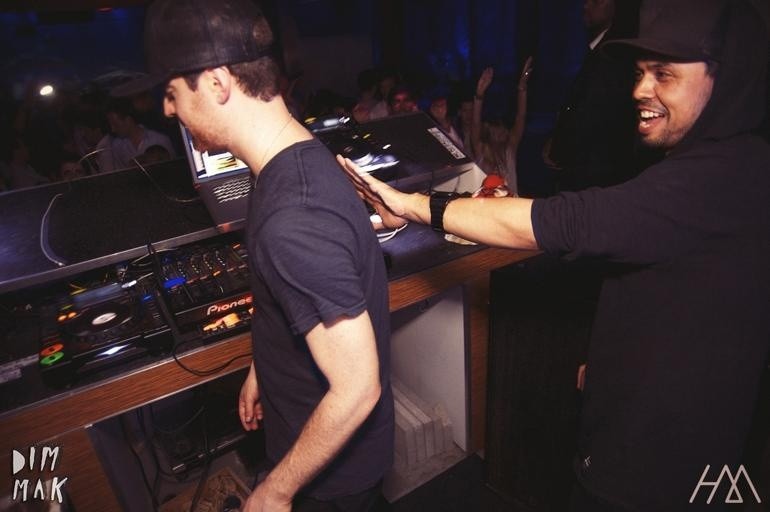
[428,190,464,232]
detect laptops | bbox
[179,120,254,227]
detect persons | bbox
[516,0,651,306]
[108,0,398,510]
[1,53,536,195]
[334,0,770,512]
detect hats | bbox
[110,0,273,97]
[602,0,721,57]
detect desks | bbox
[1,167,542,512]
